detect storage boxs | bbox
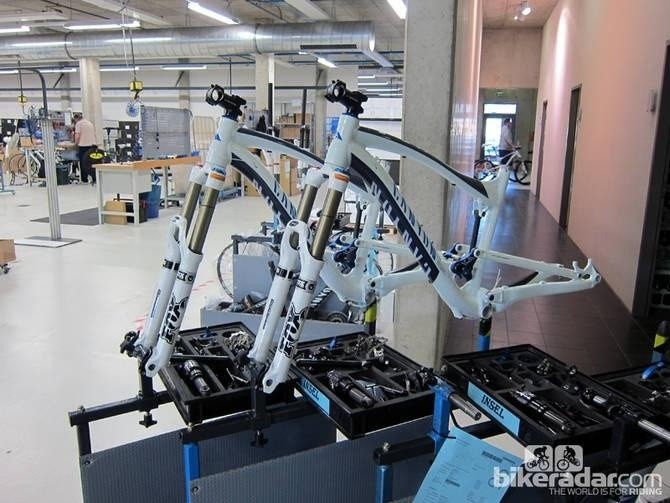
[104,201,133,226]
[243,113,314,197]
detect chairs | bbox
[61,150,80,184]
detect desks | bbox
[36,144,77,150]
[92,157,200,225]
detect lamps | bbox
[129,28,143,91]
[0,0,408,74]
[515,2,532,22]
[17,60,28,103]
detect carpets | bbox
[30,207,99,226]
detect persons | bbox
[36,112,104,185]
[499,118,520,166]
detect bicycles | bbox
[9,145,82,185]
[474,143,532,185]
